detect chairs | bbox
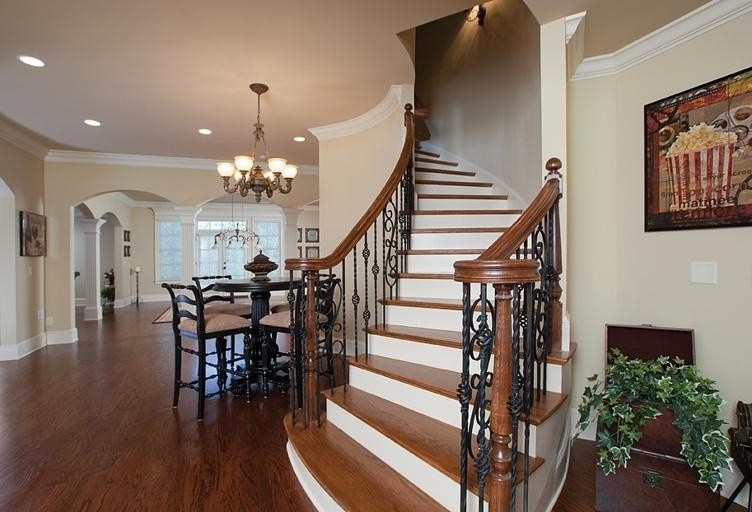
[269,273,336,362]
[258,278,340,407]
[192,275,251,386]
[722,399,752,512]
[162,282,251,421]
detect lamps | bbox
[216,82,298,203]
[211,196,260,249]
[134,266,141,304]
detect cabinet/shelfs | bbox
[595,441,720,512]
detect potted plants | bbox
[571,347,733,492]
[100,287,114,313]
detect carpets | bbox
[152,303,180,324]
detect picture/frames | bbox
[21,211,47,257]
[296,228,302,242]
[305,228,319,242]
[644,67,752,231]
[124,246,130,256]
[297,246,302,258]
[124,230,130,241]
[306,246,319,258]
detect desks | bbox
[213,276,336,393]
